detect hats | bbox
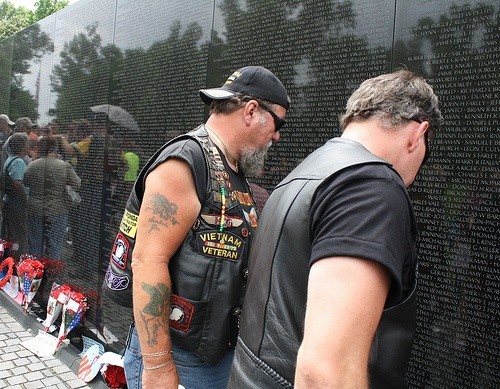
[199,66,291,110]
[0,113,15,125]
[16,116,35,126]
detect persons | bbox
[0,113,148,280]
[102,65,290,389]
[2,132,30,260]
[226,70,442,389]
[24,136,81,262]
[121,140,140,209]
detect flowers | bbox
[0,238,89,355]
[76,335,127,389]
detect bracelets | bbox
[142,348,173,358]
[60,136,66,141]
[143,359,173,371]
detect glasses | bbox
[242,97,288,132]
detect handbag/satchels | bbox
[1,157,17,196]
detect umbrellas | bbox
[90,104,141,134]
